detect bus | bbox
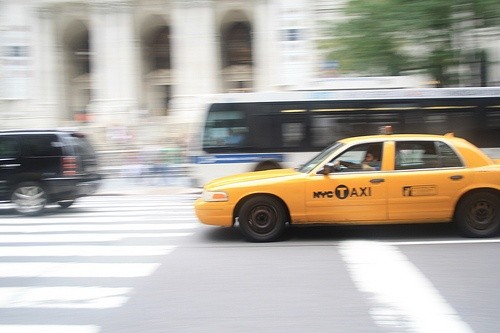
[189,77,499,193]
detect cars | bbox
[196,126,500,243]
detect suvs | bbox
[0,129,104,219]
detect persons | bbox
[213,128,244,146]
[334,150,380,171]
